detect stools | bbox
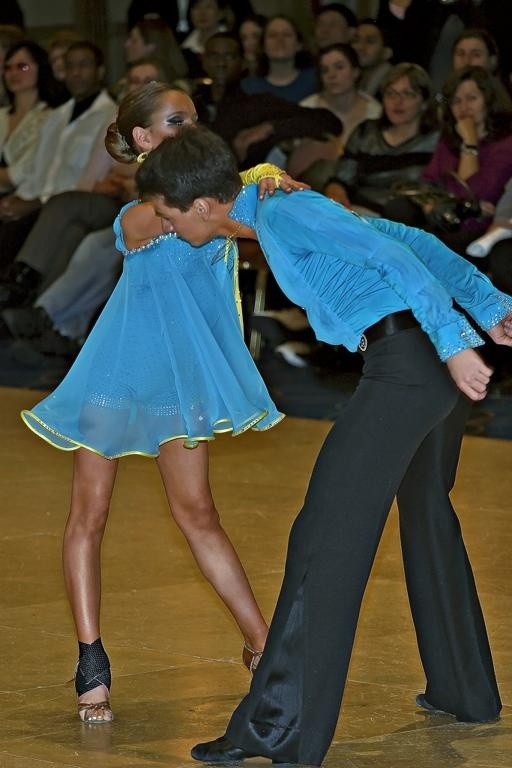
[237,240,271,360]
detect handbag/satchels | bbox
[382,170,484,234]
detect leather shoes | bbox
[191,735,321,767]
[416,694,500,724]
[358,310,420,345]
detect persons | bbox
[134,122,512,765]
[19,81,310,725]
[1,0,511,439]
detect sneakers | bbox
[248,309,316,341]
[275,343,339,370]
[10,328,80,369]
[0,307,54,340]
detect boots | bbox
[0,261,44,309]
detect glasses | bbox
[204,52,241,63]
[380,86,421,101]
[128,78,153,87]
[4,60,41,74]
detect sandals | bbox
[242,646,263,677]
[74,656,114,725]
